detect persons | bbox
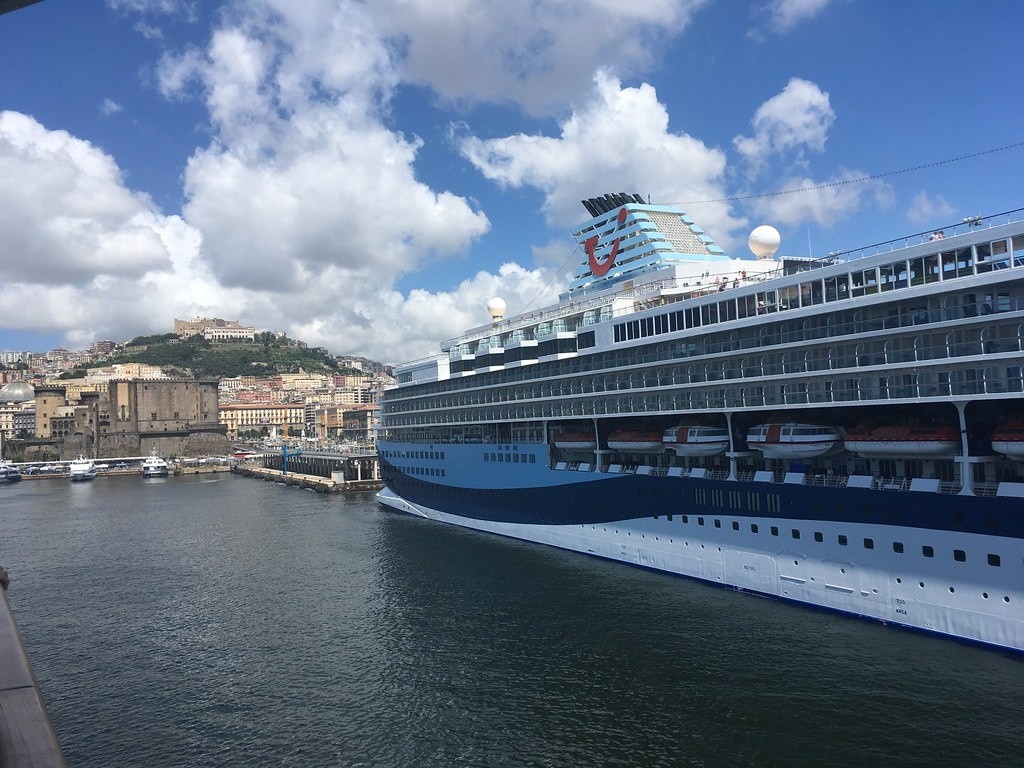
[719,284,724,292]
[733,278,739,288]
[742,269,746,282]
[930,231,944,241]
[660,297,665,306]
[715,276,720,283]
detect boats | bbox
[744,420,845,459]
[0,466,22,483]
[660,425,729,457]
[842,425,961,455]
[554,431,597,450]
[606,422,662,452]
[991,424,1024,461]
[69,459,97,481]
[140,455,168,478]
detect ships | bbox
[374,191,1024,648]
